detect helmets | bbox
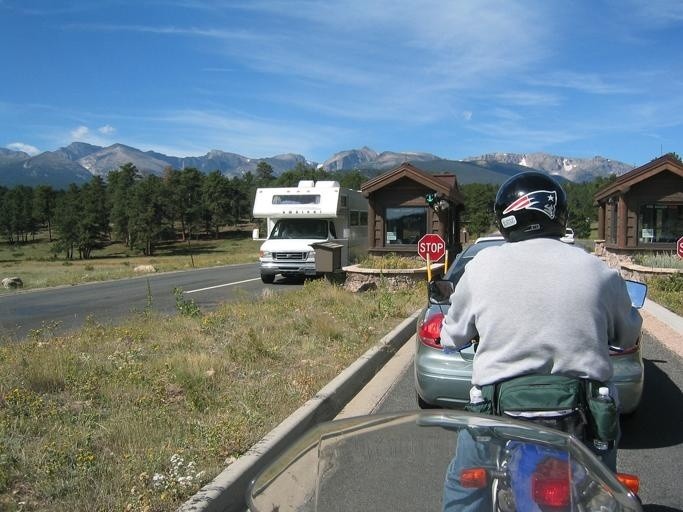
[493,171,566,242]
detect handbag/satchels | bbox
[465,372,620,468]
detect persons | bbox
[437,172,642,511]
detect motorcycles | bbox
[431,272,640,512]
